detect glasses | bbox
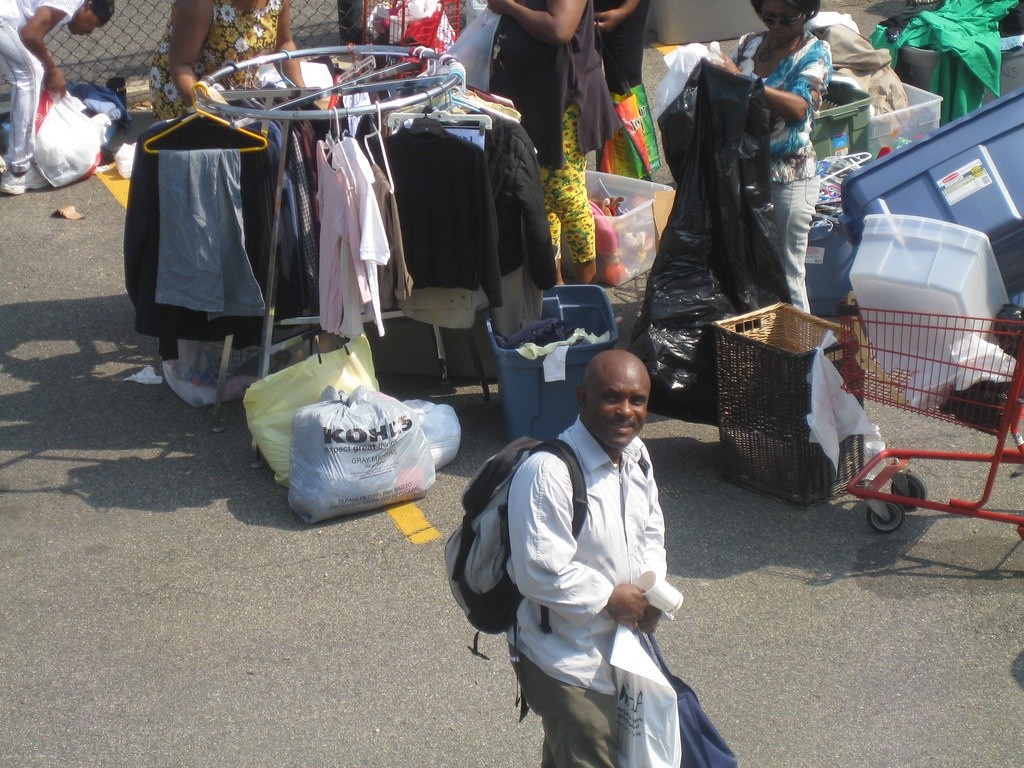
[763,10,806,27]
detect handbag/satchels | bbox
[594,27,661,180]
[242,325,379,487]
[32,79,131,187]
[290,385,437,525]
[401,397,461,470]
[609,615,680,768]
[633,623,739,768]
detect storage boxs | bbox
[849,214,1009,372]
[867,82,944,163]
[810,81,873,161]
[841,85,1024,298]
[483,285,619,444]
[559,170,674,287]
[895,34,1024,127]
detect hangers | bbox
[143,43,523,194]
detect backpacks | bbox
[444,433,589,661]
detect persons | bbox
[710,0,832,316]
[506,346,669,768]
[592,0,651,95]
[486,0,622,285]
[0,0,118,194]
[149,0,305,122]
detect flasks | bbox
[106,76,127,110]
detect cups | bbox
[636,569,684,616]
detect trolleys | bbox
[838,304,1022,547]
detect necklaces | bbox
[758,32,800,62]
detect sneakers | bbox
[2,167,49,194]
